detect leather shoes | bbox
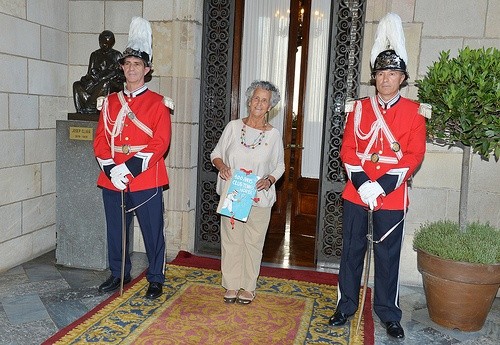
[98,275,131,293]
[328,311,355,327]
[146,282,163,299]
[381,320,404,339]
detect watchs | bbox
[263,175,272,186]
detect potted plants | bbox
[412,45,500,331]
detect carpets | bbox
[39,249,374,345]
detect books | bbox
[216,169,262,223]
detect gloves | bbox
[358,180,377,210]
[110,176,129,190]
[110,162,131,179]
[359,181,384,204]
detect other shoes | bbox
[237,292,255,305]
[224,291,239,304]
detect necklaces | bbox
[240,117,269,149]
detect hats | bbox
[116,17,154,67]
[370,12,409,81]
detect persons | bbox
[73,30,126,114]
[328,12,432,338]
[209,81,285,305]
[93,15,175,299]
